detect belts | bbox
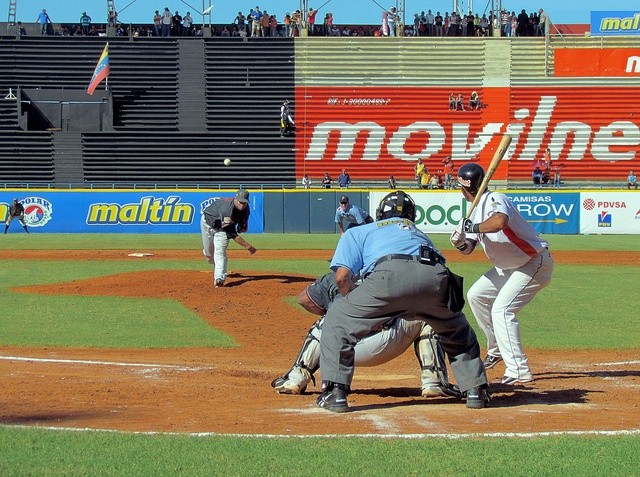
[372,254,443,269]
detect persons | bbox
[154,8,192,35]
[322,12,335,34]
[413,8,545,36]
[335,23,383,36]
[4,197,30,233]
[234,10,246,35]
[272,272,461,398]
[442,156,455,186]
[429,172,440,189]
[544,145,552,167]
[388,8,397,36]
[405,25,413,36]
[193,24,233,36]
[450,163,554,383]
[338,170,350,189]
[16,20,28,35]
[35,8,153,36]
[280,98,295,136]
[628,169,638,189]
[248,7,318,36]
[388,174,397,188]
[469,91,480,109]
[447,92,456,108]
[318,190,492,413]
[322,174,331,188]
[455,93,467,111]
[200,190,257,286]
[413,156,430,188]
[302,172,310,189]
[532,166,560,185]
[335,195,374,234]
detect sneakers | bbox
[466,387,495,408]
[482,354,503,369]
[315,382,348,412]
[490,376,533,386]
[270,378,300,395]
[422,382,462,398]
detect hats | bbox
[339,195,349,203]
[235,189,250,203]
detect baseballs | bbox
[224,158,231,165]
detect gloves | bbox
[457,217,479,233]
[450,230,468,252]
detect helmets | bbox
[457,163,488,193]
[376,191,415,222]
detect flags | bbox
[86,43,110,95]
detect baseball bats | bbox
[466,135,513,219]
